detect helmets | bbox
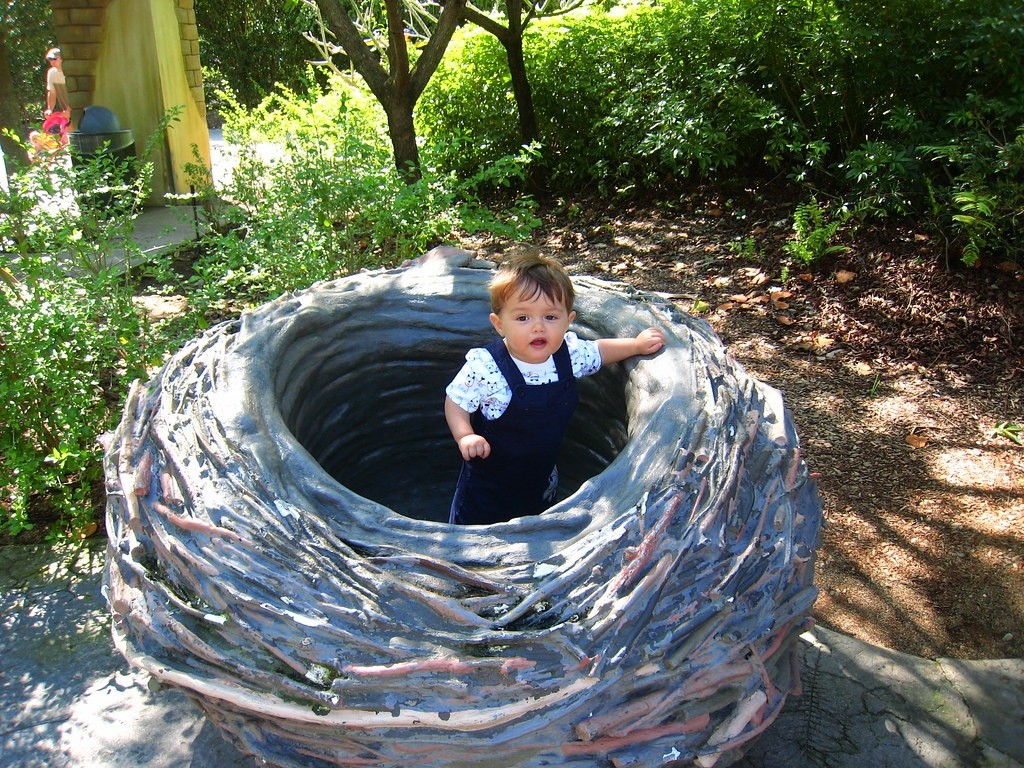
[78,106,120,132]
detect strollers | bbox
[28,108,71,164]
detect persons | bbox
[444,256,666,526]
[44,48,74,156]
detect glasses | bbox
[49,58,57,60]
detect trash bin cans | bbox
[66,106,140,218]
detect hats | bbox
[46,48,61,58]
